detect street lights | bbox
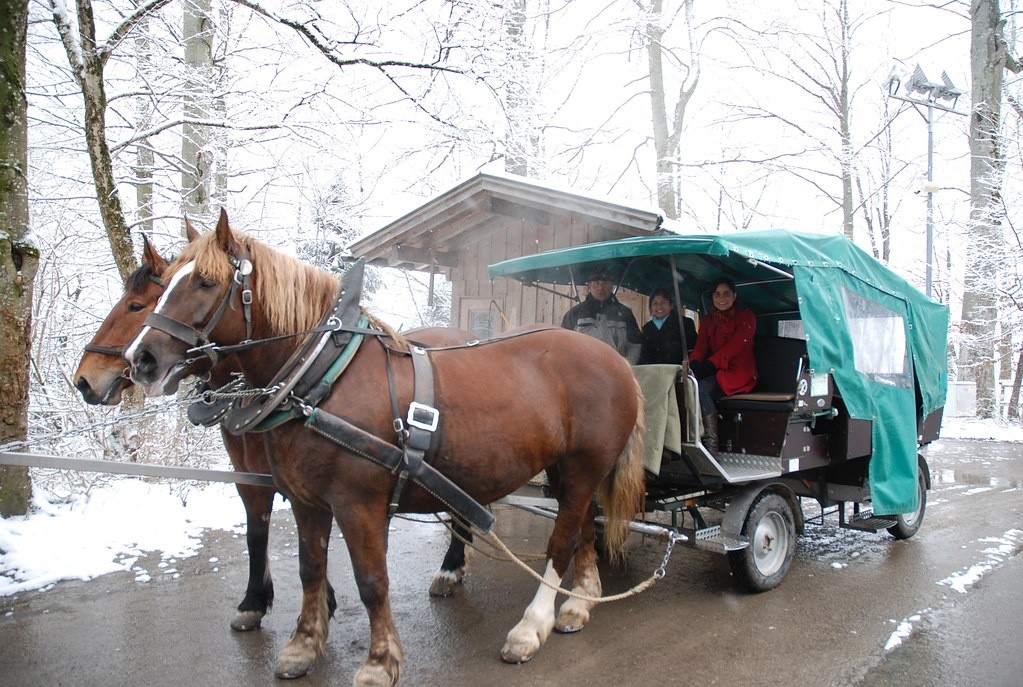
[887,62,968,299]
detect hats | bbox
[589,268,613,282]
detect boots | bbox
[701,410,720,464]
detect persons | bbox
[675,275,757,463]
[636,287,698,365]
[560,266,643,365]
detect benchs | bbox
[715,333,807,415]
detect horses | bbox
[72,205,644,687]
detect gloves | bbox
[690,359,716,380]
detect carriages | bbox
[71,204,952,687]
